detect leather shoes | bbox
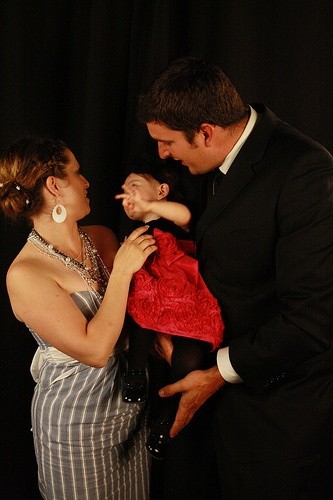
[123,366,147,402]
[146,394,179,460]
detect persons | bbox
[0,135,151,500]
[137,57,332,499]
[113,169,225,434]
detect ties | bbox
[212,170,223,194]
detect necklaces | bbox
[27,226,111,312]
